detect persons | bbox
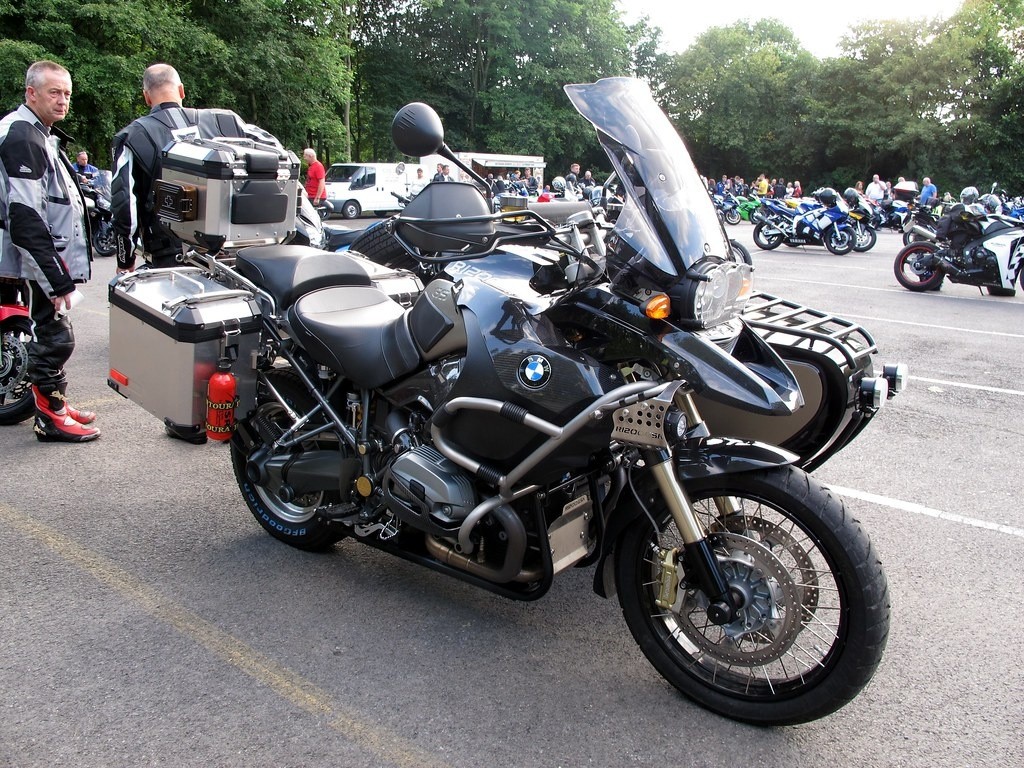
[700,174,803,198]
[71,152,99,186]
[552,176,566,197]
[485,166,539,210]
[0,61,104,443]
[432,164,455,182]
[919,177,937,204]
[578,171,596,186]
[409,167,431,202]
[566,163,580,185]
[110,63,278,273]
[543,184,551,193]
[302,148,327,208]
[855,174,906,213]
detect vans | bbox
[325,163,421,219]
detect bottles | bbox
[206,357,236,441]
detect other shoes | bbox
[166,424,208,445]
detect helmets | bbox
[844,188,860,206]
[582,187,592,199]
[552,176,566,191]
[961,187,980,204]
[820,189,838,207]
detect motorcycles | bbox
[77,170,117,257]
[706,183,760,225]
[106,75,908,728]
[0,304,39,425]
[753,188,877,256]
[870,181,1024,297]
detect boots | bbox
[65,401,96,424]
[30,383,101,442]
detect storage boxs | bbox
[892,180,919,201]
[106,268,259,432]
[156,133,301,247]
